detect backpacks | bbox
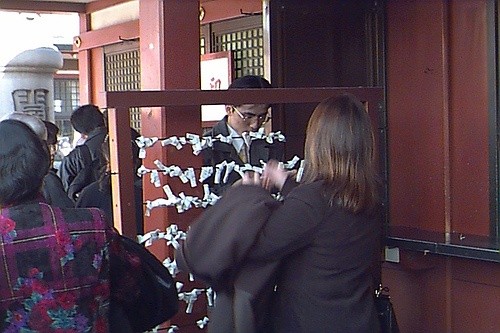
[68,145,107,203]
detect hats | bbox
[2,112,48,140]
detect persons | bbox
[202,75,284,209]
[0,104,144,246]
[241,93,384,333]
[0,119,144,333]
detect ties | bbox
[242,143,248,164]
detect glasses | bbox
[232,104,271,125]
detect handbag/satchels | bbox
[106,226,178,333]
[373,284,399,333]
